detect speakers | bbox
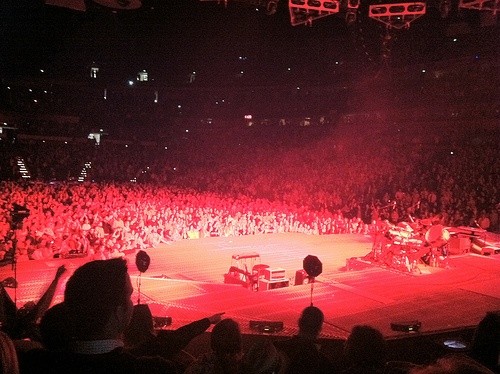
[294,269,312,286]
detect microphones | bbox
[392,202,396,210]
[418,201,420,209]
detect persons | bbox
[0,114,500,262]
[1,249,500,374]
